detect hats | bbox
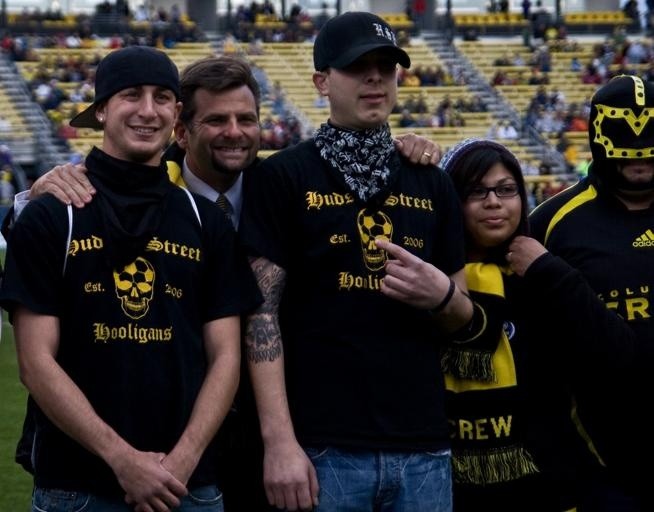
[589,74,654,175]
[440,138,530,235]
[314,12,410,71]
[70,47,181,128]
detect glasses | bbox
[469,184,519,200]
